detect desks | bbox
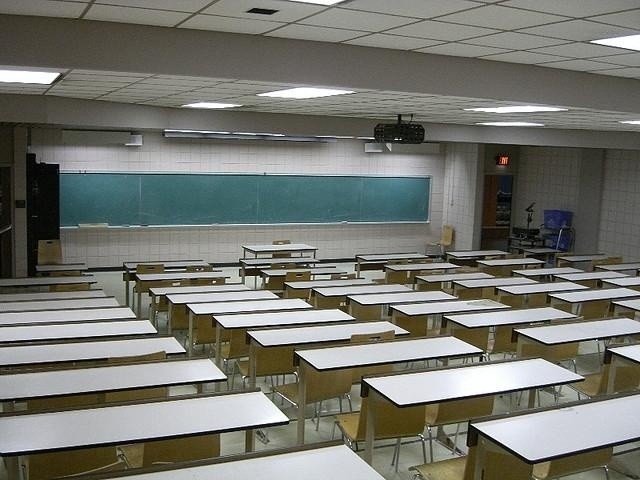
[1,386,291,479]
[462,393,639,479]
[357,359,586,469]
[2,228,639,395]
[119,439,391,479]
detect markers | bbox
[140,224,148,226]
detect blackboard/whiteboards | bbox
[59,170,433,229]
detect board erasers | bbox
[122,224,129,227]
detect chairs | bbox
[19,445,120,478]
[532,444,613,479]
[118,430,222,472]
[410,450,466,480]
[425,395,494,454]
[2,399,17,414]
[105,386,170,406]
[267,345,350,440]
[330,385,426,467]
[27,390,102,411]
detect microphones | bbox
[525,202,535,211]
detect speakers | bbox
[365,143,383,152]
[124,135,143,146]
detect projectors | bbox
[374,123,425,144]
